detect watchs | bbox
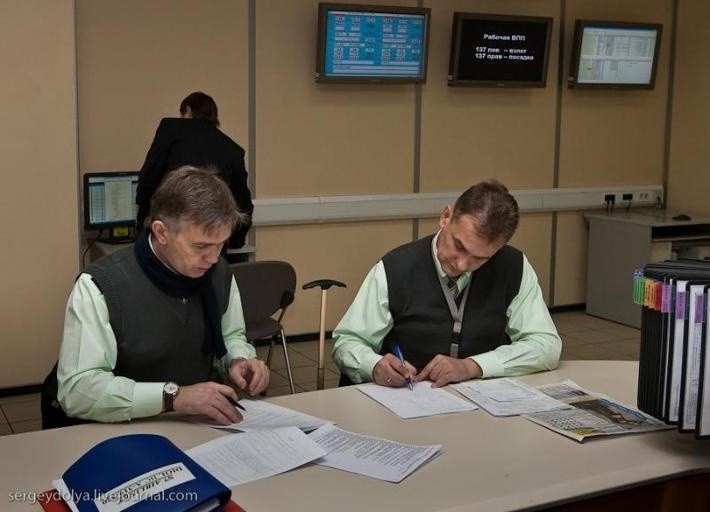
[163,382,181,413]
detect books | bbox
[629,256,710,439]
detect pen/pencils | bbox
[394,343,413,390]
[208,378,248,413]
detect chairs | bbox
[227,260,296,389]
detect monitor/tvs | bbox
[447,12,553,89]
[83,170,140,231]
[315,2,431,85]
[567,19,663,91]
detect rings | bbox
[385,375,393,383]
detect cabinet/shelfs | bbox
[585,210,710,333]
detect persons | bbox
[134,90,254,257]
[329,177,564,390]
[43,165,272,426]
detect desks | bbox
[2,358,710,512]
